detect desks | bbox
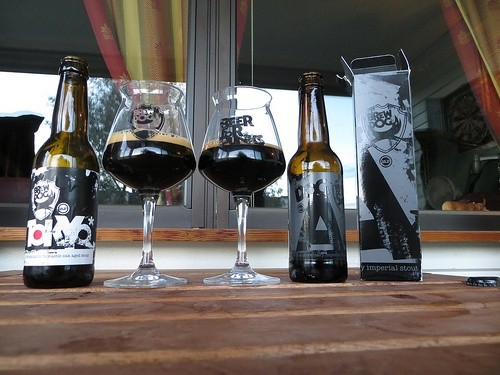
[0,268,500,375]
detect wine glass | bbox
[102,81,197,288]
[198,85,285,286]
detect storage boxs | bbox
[335,48,423,282]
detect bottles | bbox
[23,56,99,288]
[287,72,348,283]
